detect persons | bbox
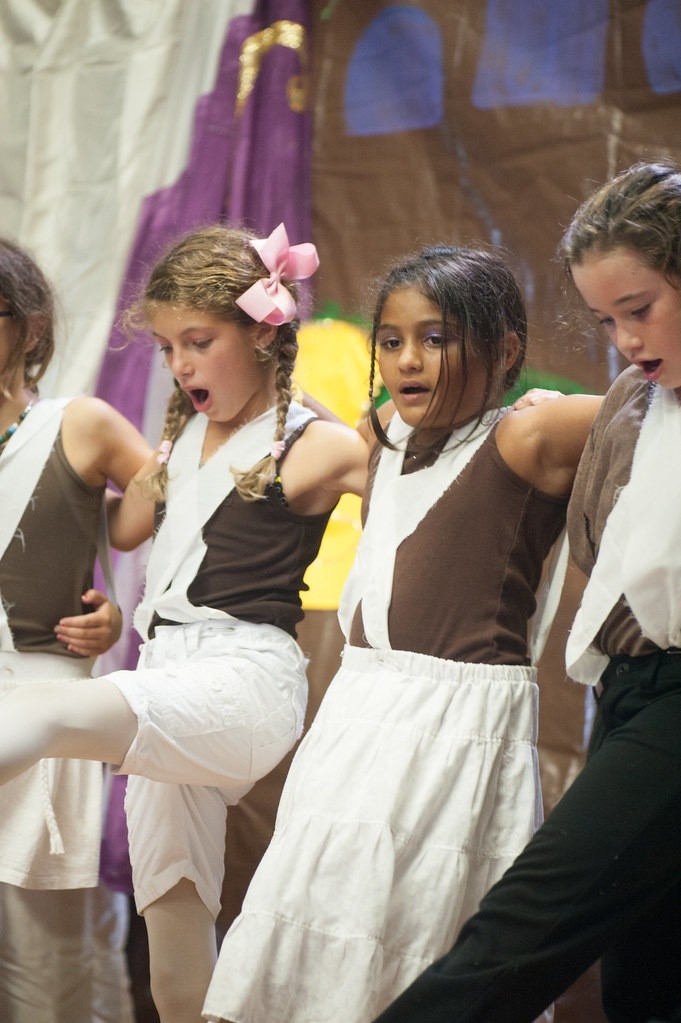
[0,223,563,1023]
[54,589,124,657]
[0,238,155,1022]
[366,160,681,1023]
[201,244,608,1023]
